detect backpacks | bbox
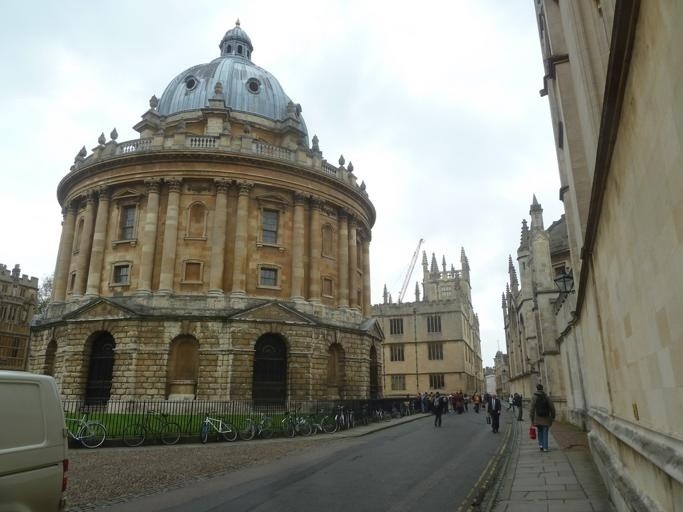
[534,393,549,416]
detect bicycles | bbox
[240,412,274,440]
[64,410,107,449]
[355,404,384,426]
[200,411,238,443]
[122,411,181,445]
[283,406,356,437]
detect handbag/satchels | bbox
[530,427,536,439]
[487,416,490,424]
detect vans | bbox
[1,370,68,512]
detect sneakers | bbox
[539,445,548,452]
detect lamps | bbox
[553,267,575,295]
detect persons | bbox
[528,384,556,453]
[404,391,523,433]
